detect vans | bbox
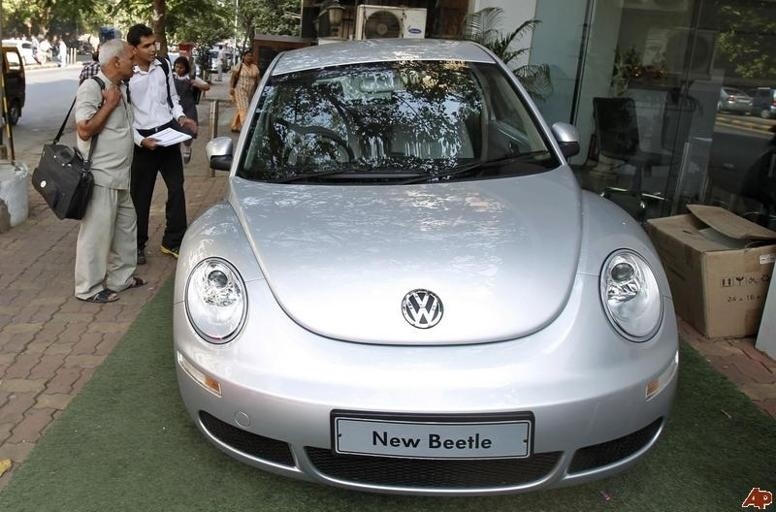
[745,87,776,120]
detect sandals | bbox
[86,289,118,304]
[128,276,146,287]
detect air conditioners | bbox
[355,5,428,40]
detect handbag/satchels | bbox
[232,73,239,88]
[31,143,93,221]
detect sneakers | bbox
[184,149,190,164]
[160,244,179,259]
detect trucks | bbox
[0,45,26,125]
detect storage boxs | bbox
[647,204,776,338]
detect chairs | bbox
[386,84,474,159]
[593,97,677,222]
[277,87,362,166]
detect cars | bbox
[716,88,753,117]
[208,42,241,72]
[173,34,681,495]
[167,52,181,71]
[3,38,46,67]
[69,41,93,56]
[191,55,201,79]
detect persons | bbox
[229,51,260,133]
[127,24,198,264]
[171,57,210,163]
[75,39,146,303]
[79,52,100,88]
